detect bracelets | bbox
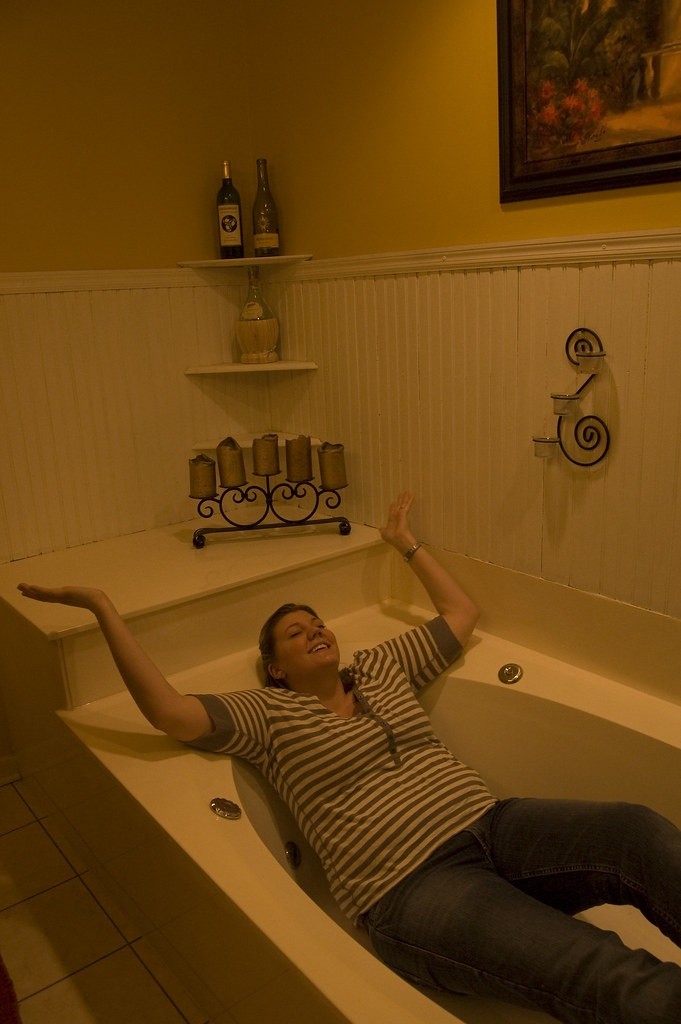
[403,542,421,562]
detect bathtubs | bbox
[56,596,681,1024]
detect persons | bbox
[17,490,681,1024]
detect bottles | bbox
[216,159,244,259]
[252,158,281,258]
[235,267,279,364]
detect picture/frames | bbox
[495,0,681,205]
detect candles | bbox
[216,436,246,488]
[252,434,279,475]
[318,442,349,489]
[285,435,313,482]
[188,452,216,497]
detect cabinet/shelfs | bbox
[177,253,322,452]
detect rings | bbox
[399,507,404,509]
